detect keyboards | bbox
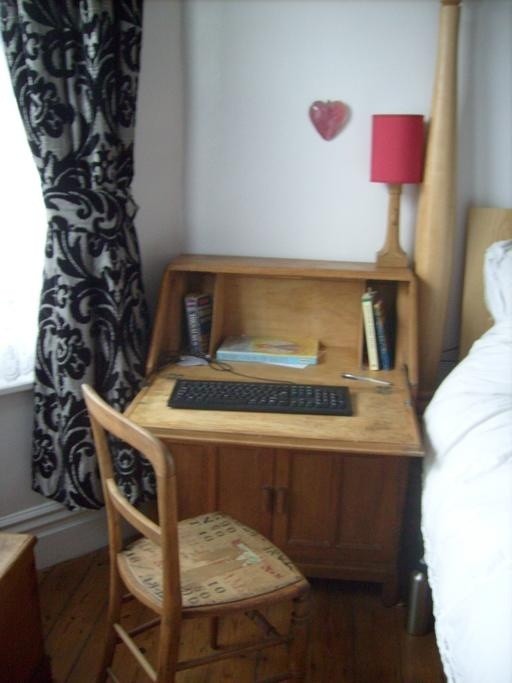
[167,379,353,417]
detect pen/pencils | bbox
[342,373,393,385]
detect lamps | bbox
[369,112,424,270]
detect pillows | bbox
[481,241,512,344]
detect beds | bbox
[418,207,512,683]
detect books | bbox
[217,330,320,370]
[183,292,212,354]
[360,287,392,371]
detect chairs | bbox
[79,382,311,683]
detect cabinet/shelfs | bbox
[0,529,55,683]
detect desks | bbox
[115,254,426,606]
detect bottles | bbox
[406,564,429,634]
